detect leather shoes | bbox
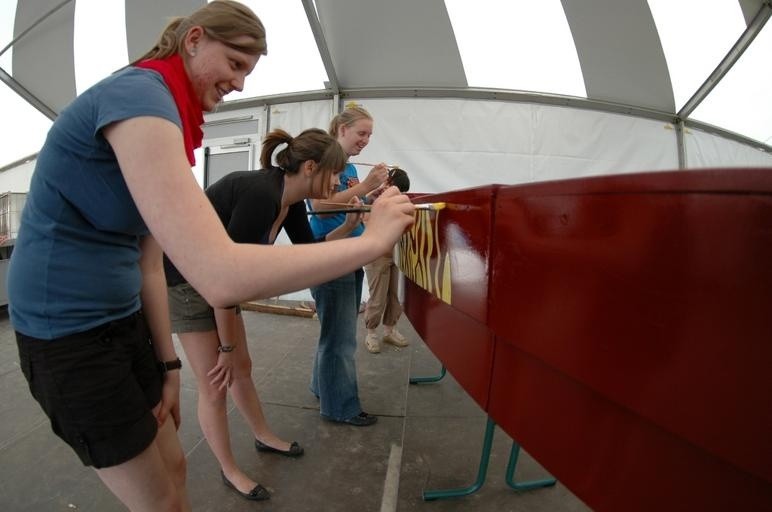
[220,470,271,501]
[344,412,377,426]
[254,438,304,457]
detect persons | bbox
[369,169,412,355]
[7,1,416,512]
[163,126,366,501]
[310,104,391,426]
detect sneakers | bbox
[365,336,380,353]
[383,328,408,346]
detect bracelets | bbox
[217,344,238,355]
[156,359,184,373]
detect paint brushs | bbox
[322,202,373,207]
[305,202,446,215]
[346,162,400,169]
